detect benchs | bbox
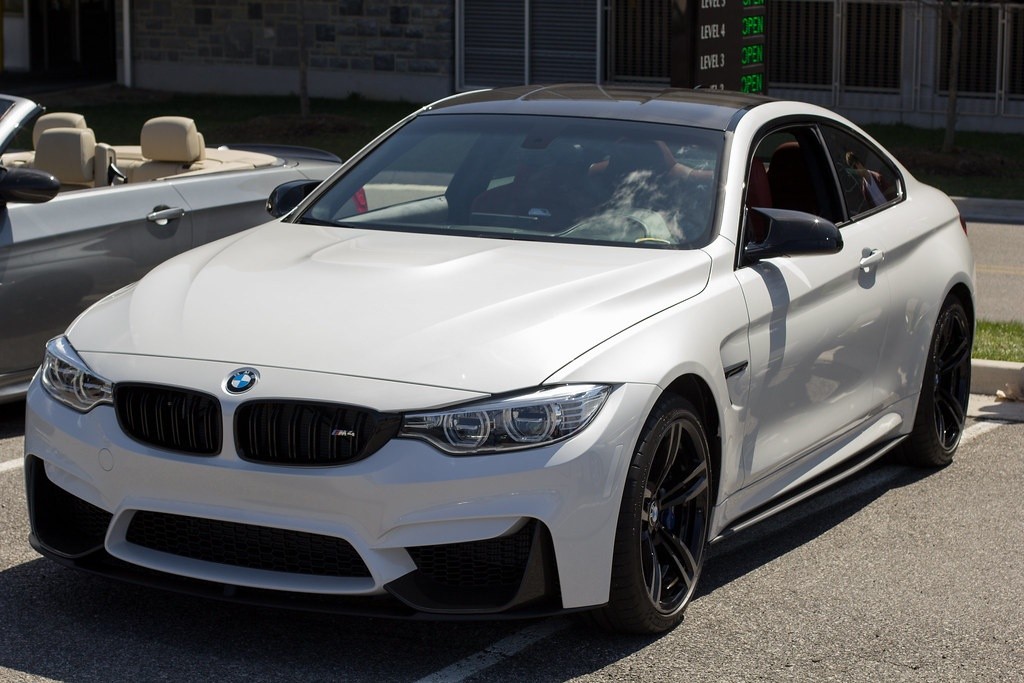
[7,114,222,182]
[583,138,866,222]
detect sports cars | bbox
[0,93,370,407]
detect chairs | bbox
[34,127,129,193]
[472,143,599,233]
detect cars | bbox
[21,79,979,634]
